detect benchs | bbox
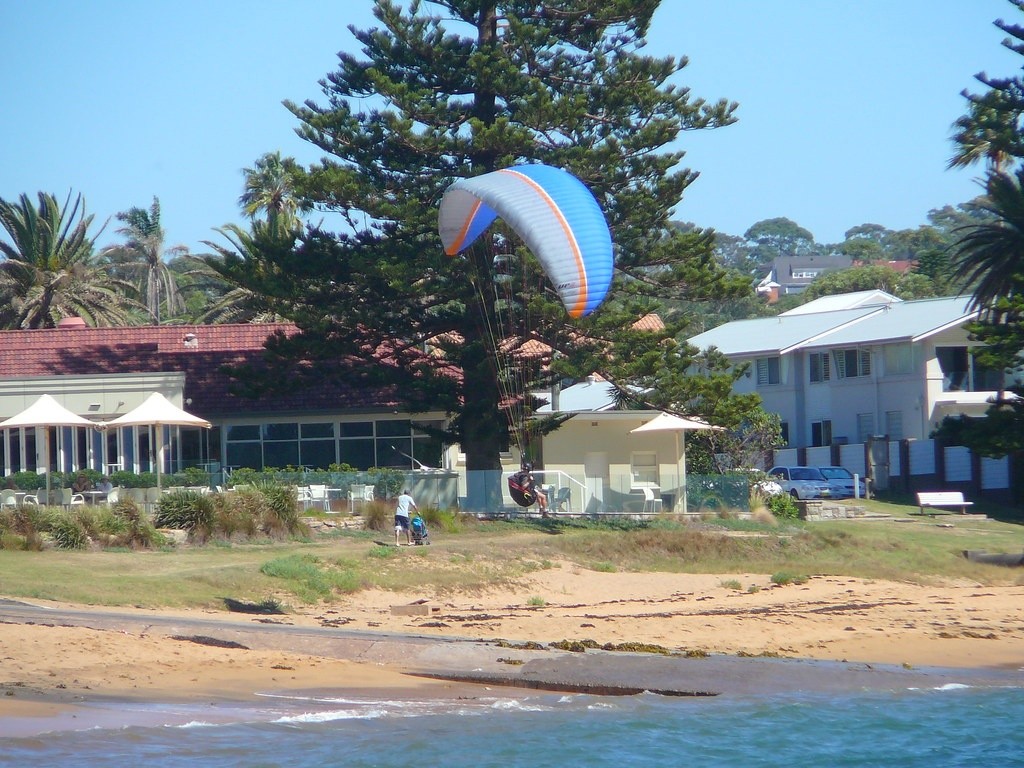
[916,491,972,515]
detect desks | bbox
[0,493,25,504]
[74,491,108,506]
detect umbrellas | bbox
[103,392,213,499]
[0,393,108,507]
[627,411,727,513]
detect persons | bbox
[88,476,113,505]
[514,460,553,518]
[73,476,91,501]
[8,479,19,490]
[394,489,421,547]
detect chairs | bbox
[23,488,85,512]
[542,484,571,513]
[129,485,230,513]
[233,484,249,493]
[98,486,120,507]
[290,484,375,513]
[1,488,16,510]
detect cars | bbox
[818,466,866,499]
[767,466,828,499]
[729,467,783,499]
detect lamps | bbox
[642,486,663,513]
[90,403,101,407]
[116,400,124,409]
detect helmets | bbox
[524,463,532,471]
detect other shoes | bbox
[543,514,550,518]
[408,542,415,546]
[540,508,543,512]
[396,544,399,547]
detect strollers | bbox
[409,514,430,545]
